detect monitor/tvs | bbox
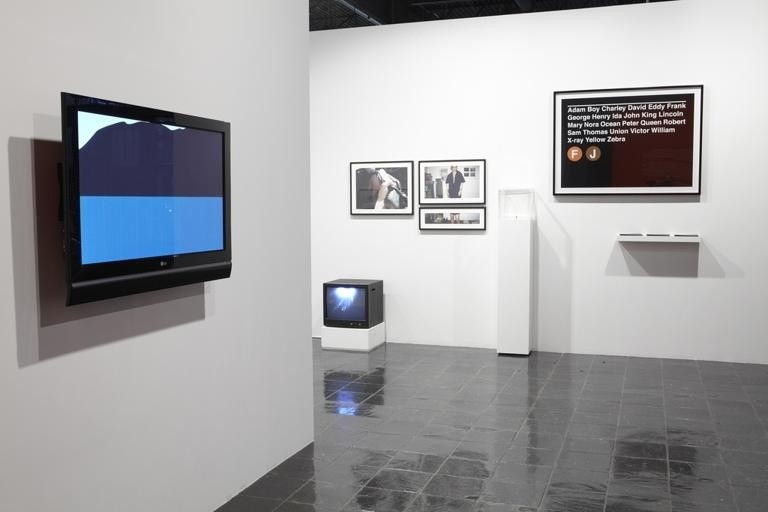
[323,280,383,329]
[61,94,232,308]
